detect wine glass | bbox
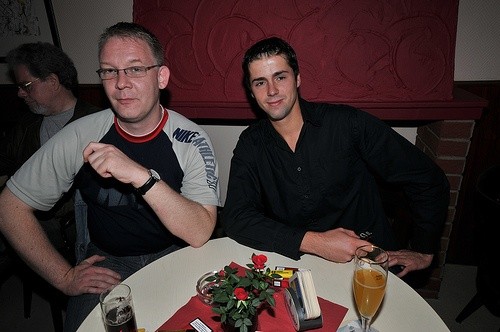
[339,246,390,332]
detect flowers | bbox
[206,254,291,331]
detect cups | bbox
[99,283,137,332]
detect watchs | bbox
[134,169,161,196]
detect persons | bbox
[1,22,222,331]
[219,37,448,279]
[5,41,105,269]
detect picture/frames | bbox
[0,0,63,64]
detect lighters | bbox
[259,270,294,278]
[274,266,298,274]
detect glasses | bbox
[17,78,40,91]
[96,65,157,80]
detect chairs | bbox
[0,83,170,332]
[456,166,500,323]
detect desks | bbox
[75,236,452,332]
